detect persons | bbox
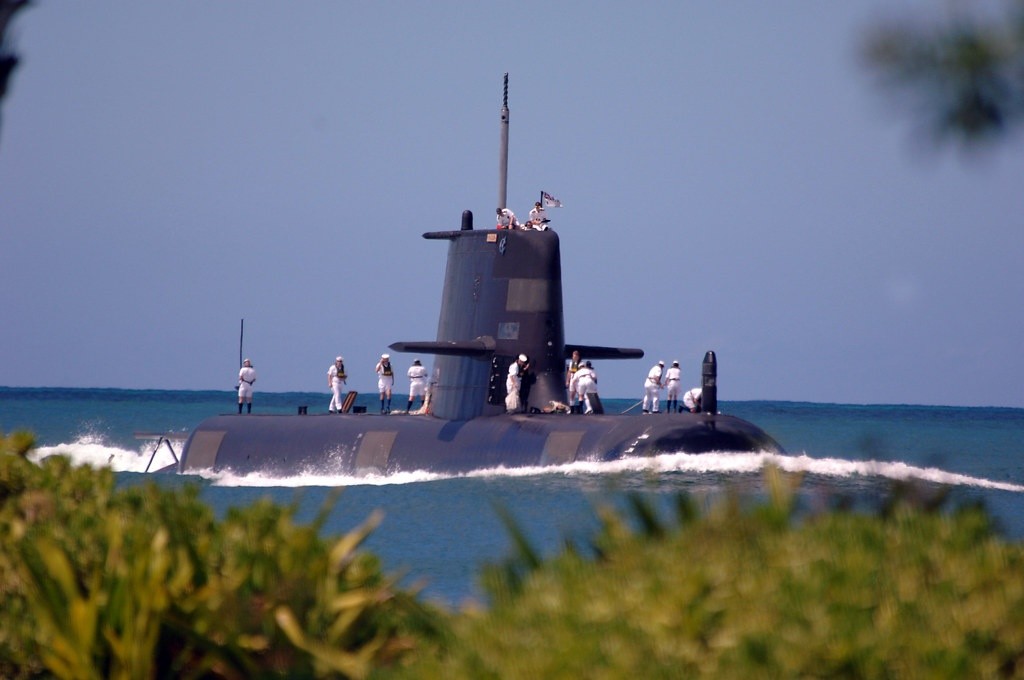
[327,357,347,414]
[642,361,664,415]
[565,351,597,414]
[506,354,530,415]
[496,202,548,231]
[666,361,681,413]
[407,359,427,411]
[679,388,702,413]
[375,353,394,413]
[238,359,256,414]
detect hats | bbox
[659,360,665,367]
[414,358,421,362]
[243,359,250,363]
[577,361,585,368]
[672,360,679,365]
[336,357,343,361]
[381,353,390,359]
[519,353,528,362]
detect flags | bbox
[542,191,562,207]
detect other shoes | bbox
[381,407,392,413]
[404,411,407,415]
